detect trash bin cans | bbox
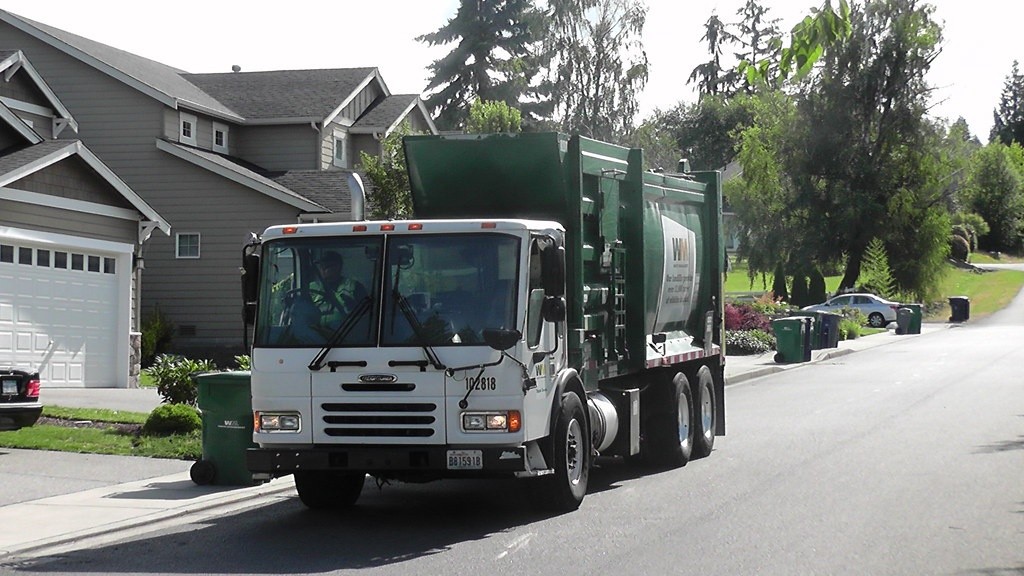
[188,368,270,488]
[948,296,970,322]
[790,310,845,349]
[788,315,812,362]
[896,303,923,334]
[772,317,806,363]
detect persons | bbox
[308,251,370,344]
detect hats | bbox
[313,251,342,265]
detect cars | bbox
[799,292,904,329]
[0,369,43,431]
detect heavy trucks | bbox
[237,127,732,515]
